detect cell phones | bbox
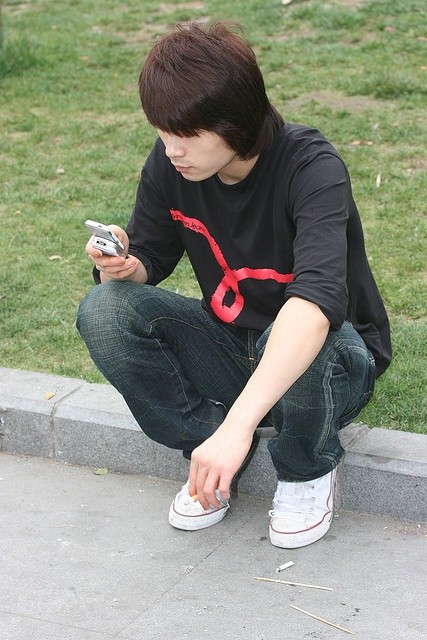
[83,220,131,259]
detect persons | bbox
[75,23,393,550]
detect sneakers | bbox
[167,436,258,530]
[268,465,336,549]
